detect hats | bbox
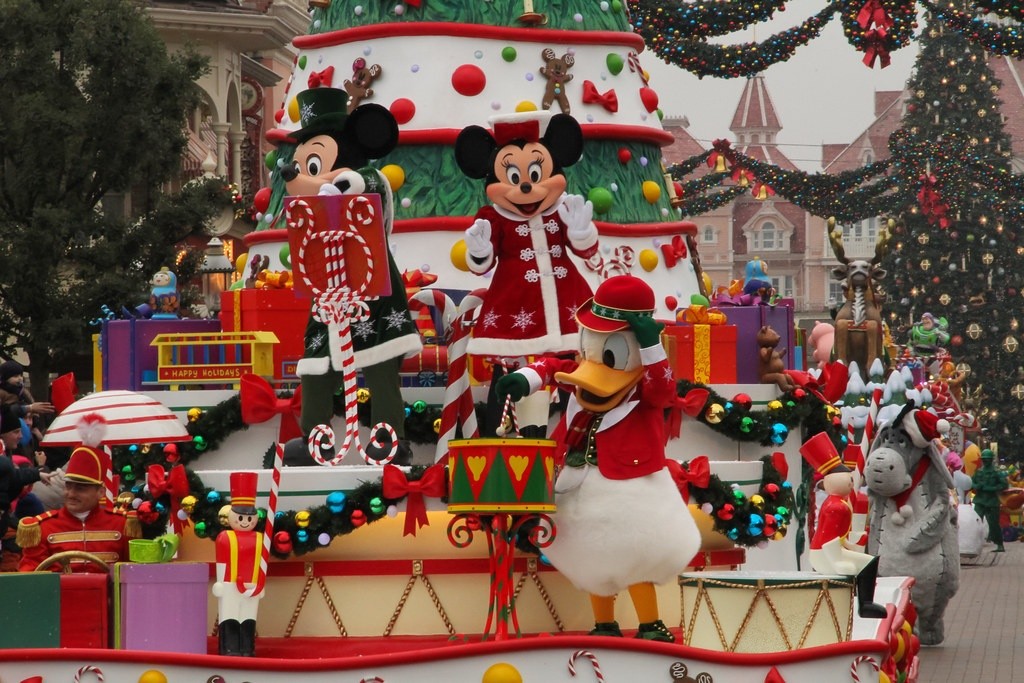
[0,403,21,434]
[0,361,23,383]
[62,413,110,484]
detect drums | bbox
[448,438,558,514]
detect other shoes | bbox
[991,548,1005,552]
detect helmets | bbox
[979,449,994,458]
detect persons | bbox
[798,431,889,619]
[971,449,1009,552]
[0,362,142,573]
[212,472,265,657]
[908,312,950,376]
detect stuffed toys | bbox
[281,85,702,643]
[864,405,961,647]
